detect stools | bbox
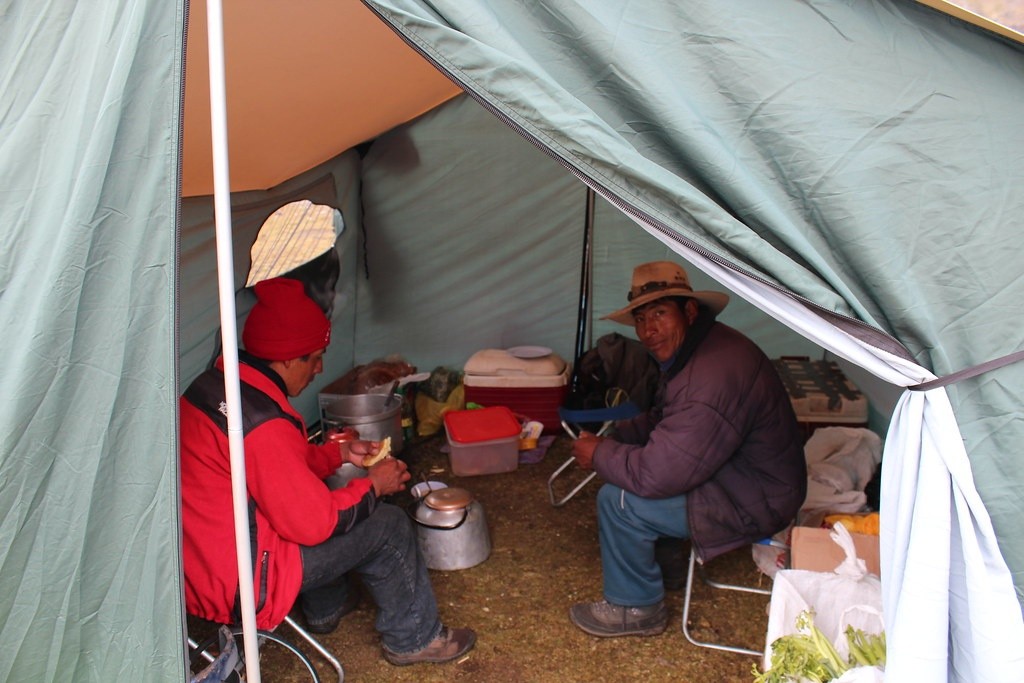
[547,402,647,508]
[188,616,344,683]
[682,509,800,658]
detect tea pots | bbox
[416,489,491,570]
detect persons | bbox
[179,278,480,669]
[567,261,810,639]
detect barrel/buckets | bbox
[326,393,402,472]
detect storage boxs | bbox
[463,348,574,437]
[443,404,523,478]
[792,524,881,583]
[776,355,870,444]
[319,365,417,443]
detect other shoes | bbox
[381,626,477,666]
[663,571,684,590]
[569,600,669,638]
[310,582,362,634]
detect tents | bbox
[0,0,1023,683]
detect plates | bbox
[507,344,550,358]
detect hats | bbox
[598,261,730,322]
[241,277,332,361]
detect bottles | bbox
[397,389,414,442]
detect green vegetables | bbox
[750,610,886,683]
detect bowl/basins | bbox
[411,481,448,496]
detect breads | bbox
[361,435,391,466]
